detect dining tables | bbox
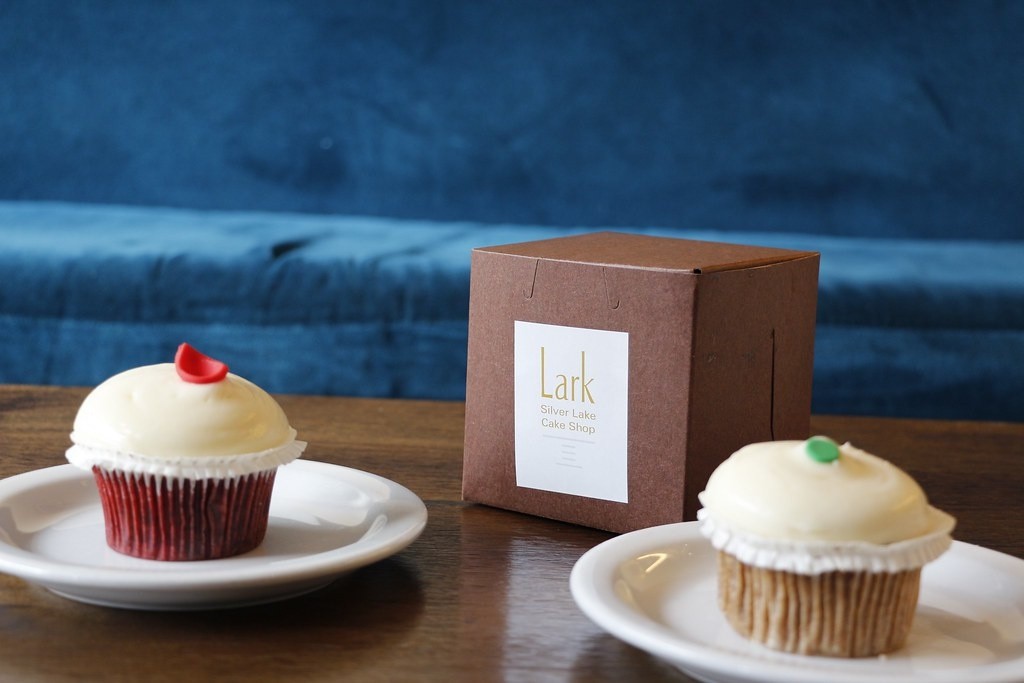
[0,383,1024,683]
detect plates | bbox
[0,458,429,611]
[569,521,1024,683]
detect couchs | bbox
[0,0,1024,414]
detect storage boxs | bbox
[460,230,821,537]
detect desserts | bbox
[696,439,954,660]
[68,342,307,560]
